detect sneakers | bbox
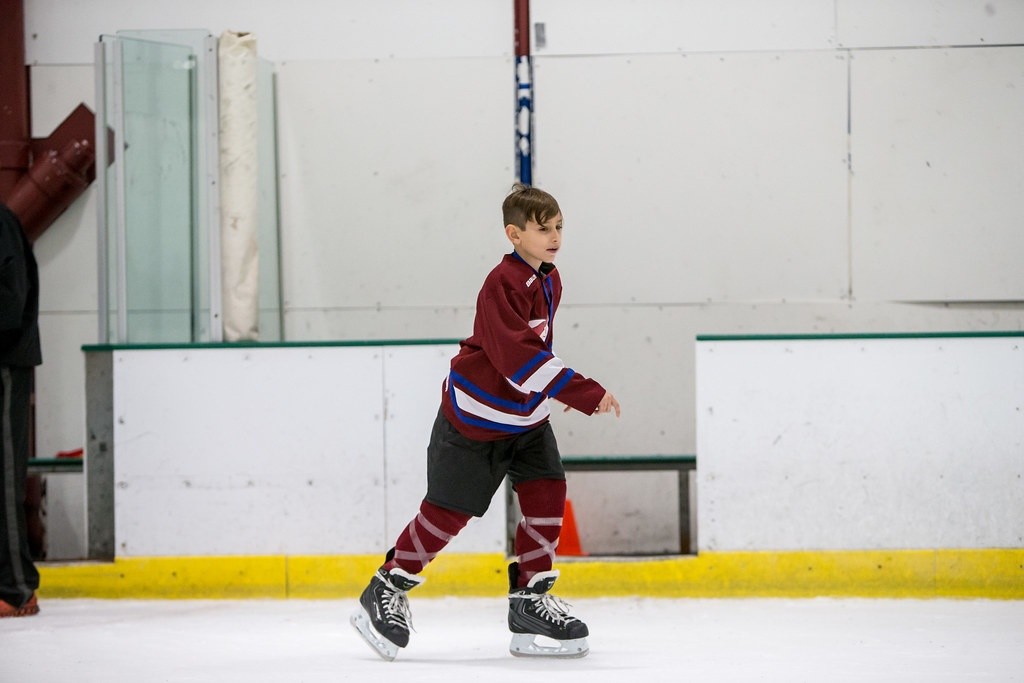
[509,562,589,659]
[351,548,423,663]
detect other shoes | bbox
[0,594,40,618]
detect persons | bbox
[351,188,620,661]
